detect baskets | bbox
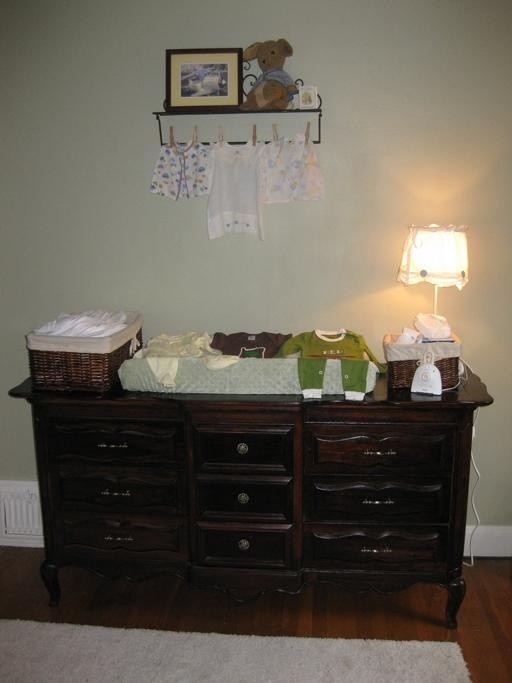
[383,331,460,392]
[24,310,143,393]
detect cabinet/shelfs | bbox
[306,400,466,625]
[8,382,189,607]
[188,395,302,608]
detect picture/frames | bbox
[166,48,243,112]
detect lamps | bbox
[396,225,469,316]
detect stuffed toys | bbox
[239,39,298,112]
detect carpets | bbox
[1,619,471,682]
